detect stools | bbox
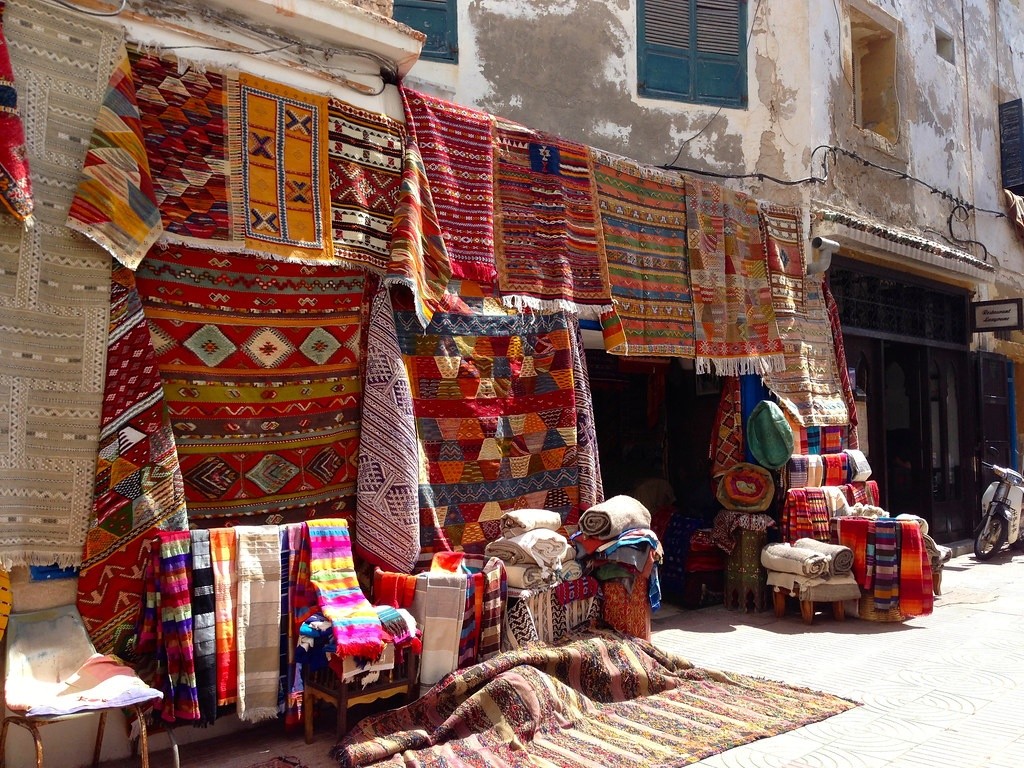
[767,570,857,624]
[931,564,944,596]
[305,643,415,745]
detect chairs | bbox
[0,604,165,768]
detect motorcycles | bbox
[973,446,1024,559]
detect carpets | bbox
[0,0,932,768]
